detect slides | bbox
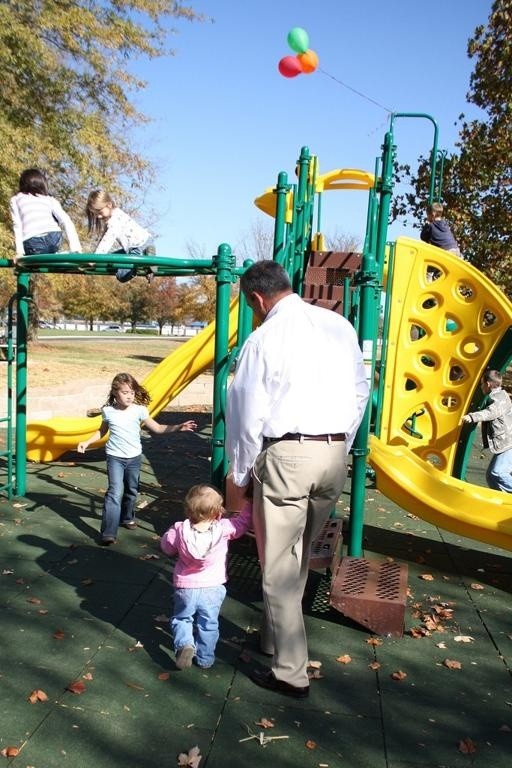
[26,294,240,462]
[367,434,512,552]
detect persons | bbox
[86,189,158,283]
[78,373,198,544]
[420,202,461,281]
[9,168,82,264]
[224,259,371,698]
[462,369,512,494]
[160,485,252,670]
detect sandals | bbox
[142,245,157,283]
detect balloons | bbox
[297,49,319,73]
[278,56,302,78]
[287,27,310,54]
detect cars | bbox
[100,324,121,332]
[36,320,60,329]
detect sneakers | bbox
[175,644,195,672]
[125,521,137,530]
[99,535,118,546]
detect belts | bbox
[263,432,346,442]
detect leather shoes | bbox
[253,631,273,658]
[249,664,309,698]
